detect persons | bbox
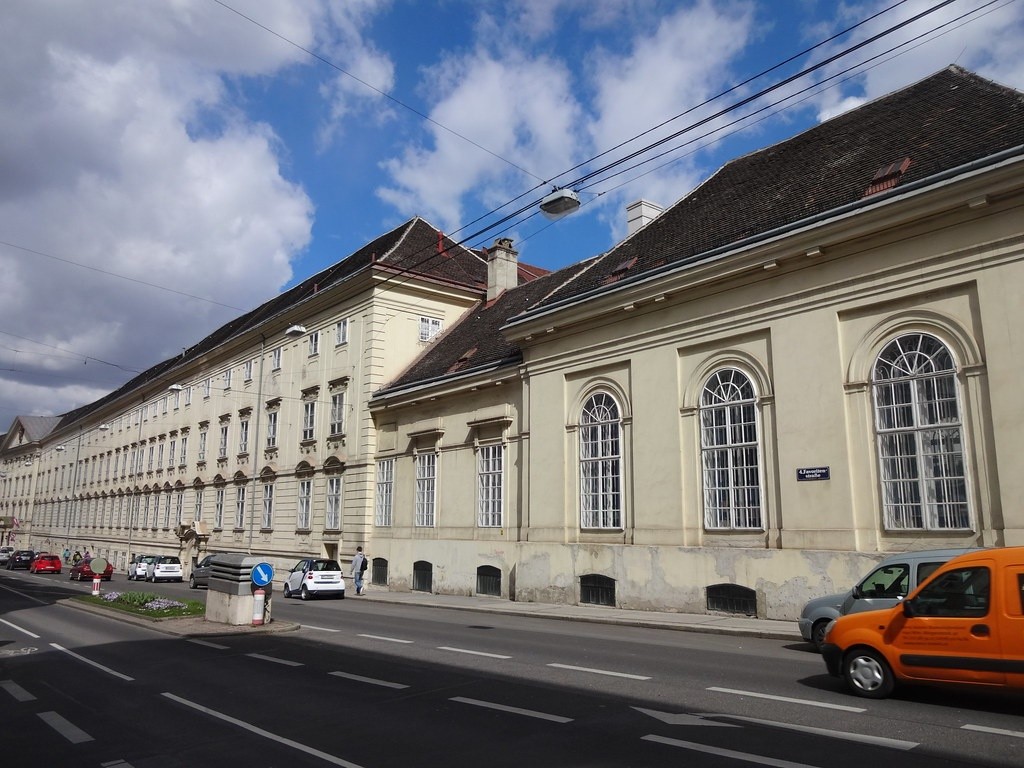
[350,546,365,596]
[63,548,71,564]
[73,551,91,564]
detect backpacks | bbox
[360,558,368,571]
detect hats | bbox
[357,547,362,551]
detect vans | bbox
[798,547,988,654]
[820,546,1024,699]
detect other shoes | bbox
[359,586,364,596]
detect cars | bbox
[69,558,113,581]
[144,556,183,583]
[0,546,15,563]
[127,555,156,581]
[29,554,62,574]
[283,558,345,601]
[5,550,34,570]
[189,554,217,589]
